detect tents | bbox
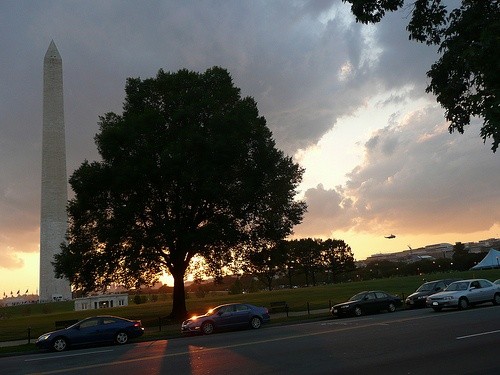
[471,248,500,270]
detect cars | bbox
[331,290,400,317]
[406,280,449,308]
[35,315,144,352]
[425,279,497,311]
[181,304,269,336]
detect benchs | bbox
[54,319,79,331]
[125,313,163,333]
[258,300,289,319]
